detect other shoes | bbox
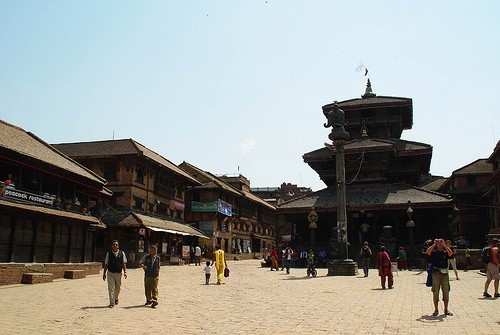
[444,309,453,316]
[483,292,492,297]
[109,305,114,308]
[151,301,158,307]
[433,309,439,316]
[145,301,151,305]
[115,300,118,305]
[494,293,500,297]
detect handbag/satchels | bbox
[224,268,229,277]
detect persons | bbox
[376,246,393,289]
[426,239,453,316]
[465,244,473,268]
[263,242,299,271]
[422,240,434,286]
[360,242,372,277]
[103,240,127,308]
[301,249,326,275]
[283,245,294,274]
[127,250,135,268]
[5,173,14,184]
[189,241,208,266]
[204,261,211,285]
[396,246,408,271]
[139,245,160,307]
[484,237,500,297]
[446,240,459,280]
[211,245,227,285]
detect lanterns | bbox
[172,236,177,241]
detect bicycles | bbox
[307,265,317,277]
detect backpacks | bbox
[362,247,370,258]
[481,246,496,264]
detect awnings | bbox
[119,212,210,239]
[0,199,101,225]
